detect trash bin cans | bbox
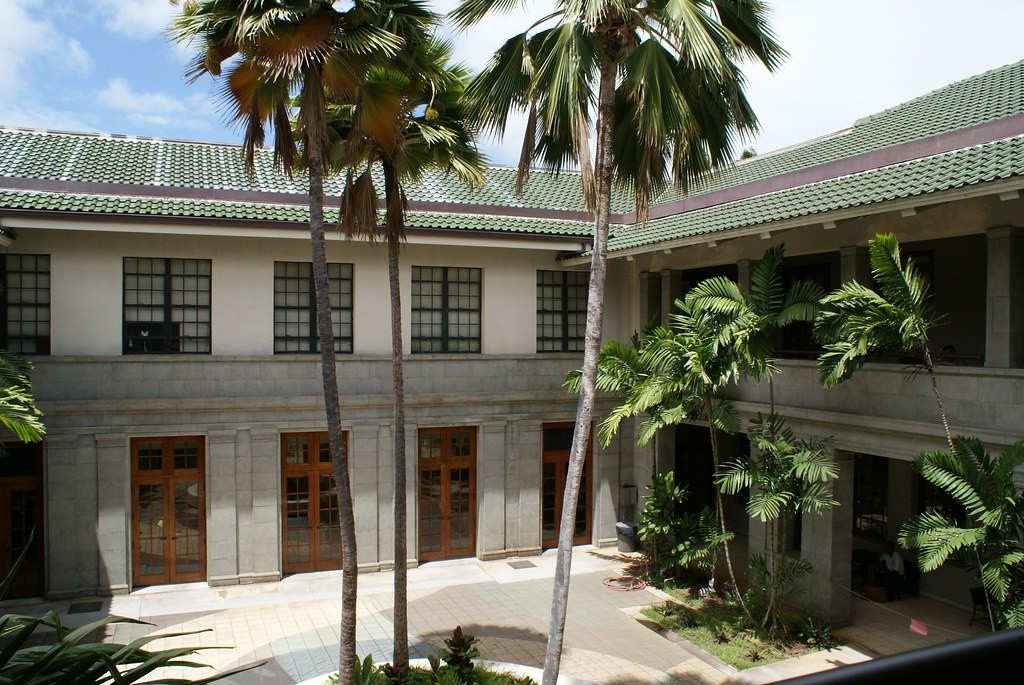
[616,522,638,552]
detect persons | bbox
[879,540,905,601]
[938,345,966,367]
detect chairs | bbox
[968,587,1004,629]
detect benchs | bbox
[853,551,921,598]
